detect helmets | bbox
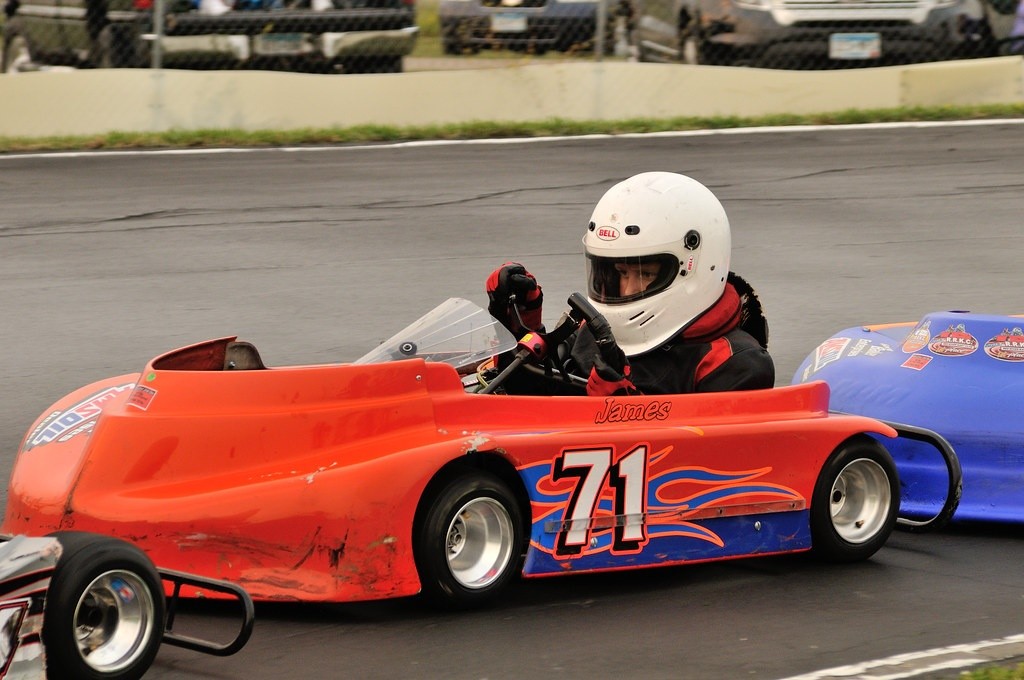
[582,170,733,358]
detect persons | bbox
[485,171,776,396]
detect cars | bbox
[1,0,419,74]
[621,1,1000,71]
[438,0,616,56]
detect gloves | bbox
[485,263,544,336]
[586,364,638,397]
